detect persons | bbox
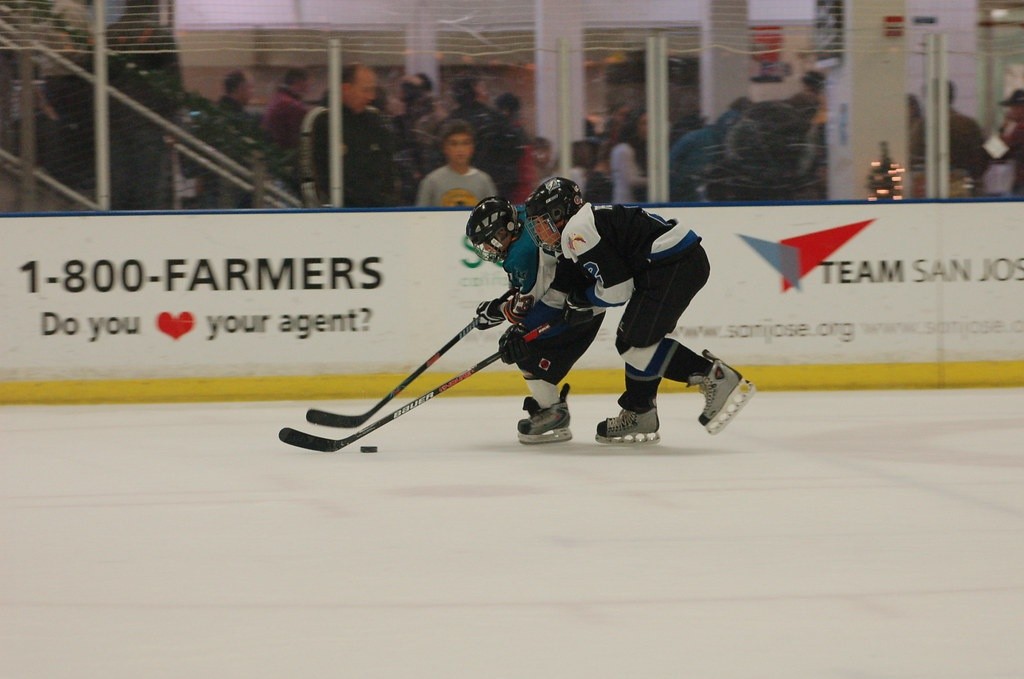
[907,79,1024,195]
[670,70,829,201]
[217,61,648,207]
[498,177,743,438]
[11,0,186,211]
[465,197,605,435]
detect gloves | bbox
[498,325,533,364]
[561,289,594,328]
[473,299,506,331]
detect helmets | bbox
[524,176,583,252]
[465,195,521,263]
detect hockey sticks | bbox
[278,311,566,453]
[304,288,518,430]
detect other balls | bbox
[360,445,378,453]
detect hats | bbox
[998,90,1024,105]
[438,118,475,143]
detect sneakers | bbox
[595,397,661,447]
[686,349,756,435]
[517,383,572,444]
